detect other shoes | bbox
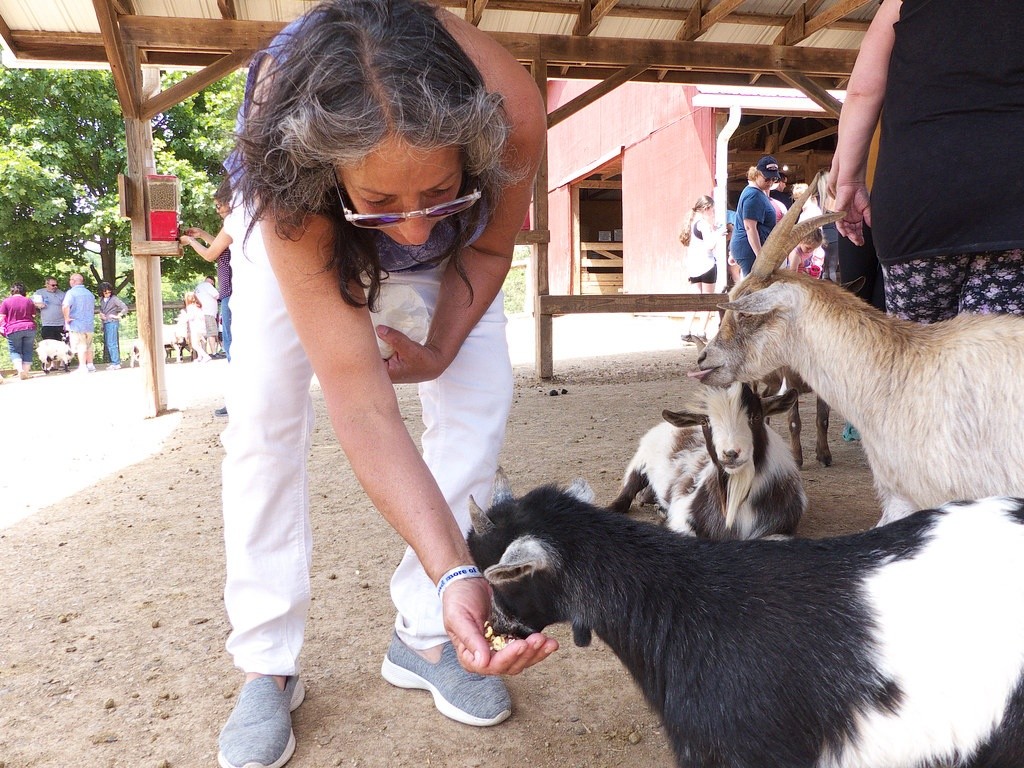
[197,354,211,363]
[19,369,30,380]
[50,364,55,371]
[212,354,226,360]
[215,407,228,417]
[682,333,708,346]
[59,365,65,371]
[88,365,96,372]
[70,367,88,374]
[842,422,861,442]
[106,362,121,370]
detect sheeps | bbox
[36,339,75,373]
[605,170,1024,540]
[465,465,1023,768]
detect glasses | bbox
[47,283,58,287]
[330,164,482,229]
[760,173,779,183]
[69,279,74,281]
[216,204,221,209]
[783,182,787,186]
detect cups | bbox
[810,266,820,277]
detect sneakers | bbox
[382,629,511,726]
[218,672,305,768]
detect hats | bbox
[756,156,781,179]
[207,276,216,283]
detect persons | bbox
[216,0,559,767]
[175,181,232,366]
[833,0,1024,338]
[98,283,129,370]
[0,283,37,380]
[727,105,887,441]
[33,273,95,374]
[680,196,728,343]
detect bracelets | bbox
[436,566,483,603]
